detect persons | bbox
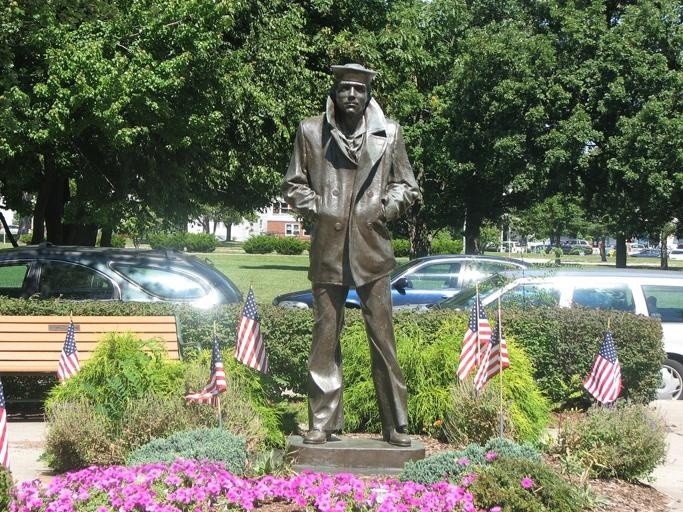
[281,62,418,448]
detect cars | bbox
[669,248,683,260]
[626,242,661,256]
[0,243,244,310]
[563,240,592,249]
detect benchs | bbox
[0,314,205,377]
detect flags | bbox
[583,330,623,406]
[0,380,10,470]
[55,317,81,383]
[456,292,494,382]
[234,286,271,375]
[472,312,510,393]
[183,334,228,408]
